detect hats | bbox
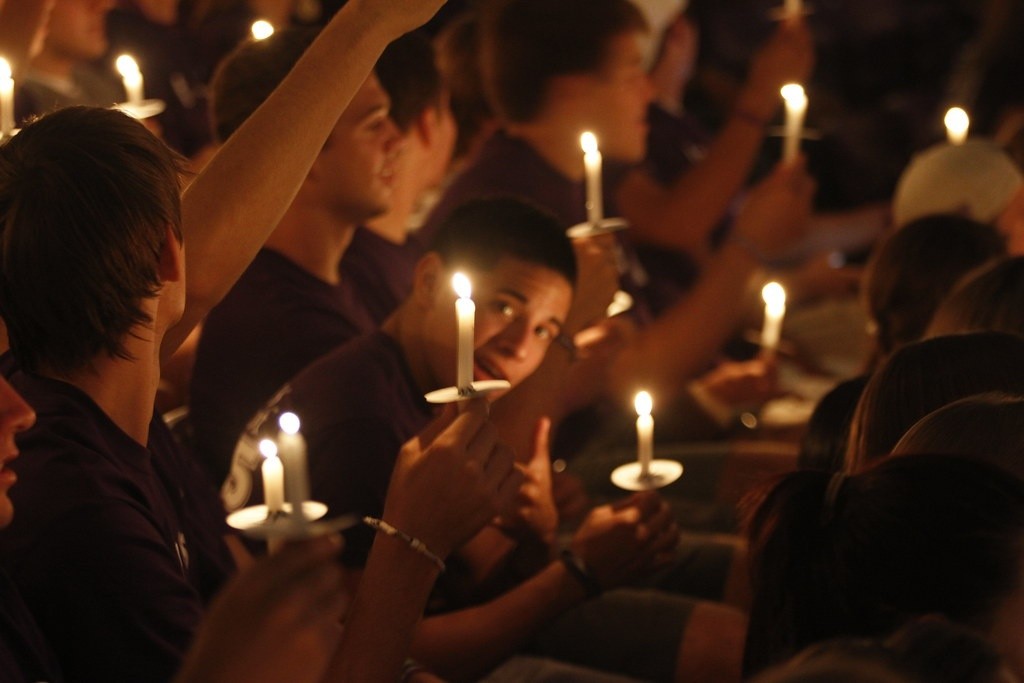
[892,140,1024,226]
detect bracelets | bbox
[552,549,603,599]
[361,513,445,572]
[398,658,427,682]
[555,333,578,361]
[727,104,769,130]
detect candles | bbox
[579,130,603,228]
[759,282,786,349]
[780,82,810,162]
[259,438,285,513]
[451,271,476,394]
[944,106,969,145]
[0,57,16,135]
[116,54,143,102]
[276,410,310,525]
[633,390,655,477]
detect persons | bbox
[750,612,1023,680]
[0,0,1024,683]
[219,196,641,682]
[1,0,447,683]
